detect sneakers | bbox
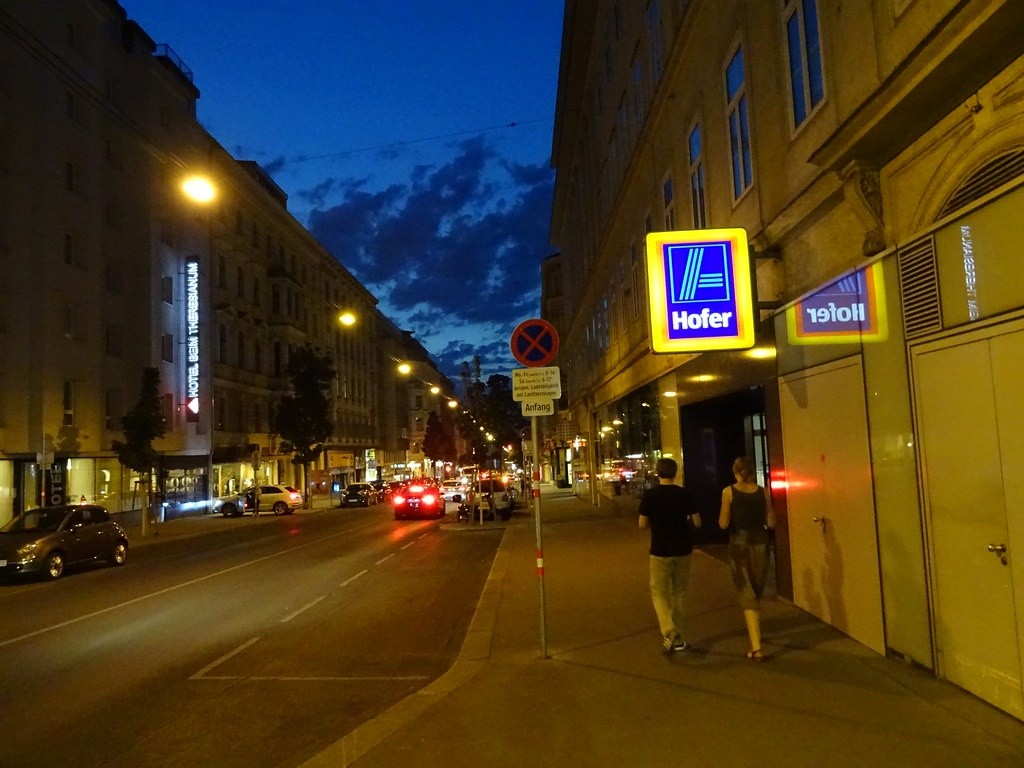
[662,632,678,654]
[675,641,687,652]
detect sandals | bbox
[745,649,763,663]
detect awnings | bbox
[212,443,260,464]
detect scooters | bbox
[457,490,503,524]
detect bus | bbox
[461,466,480,482]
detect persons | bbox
[252,484,262,517]
[520,474,526,495]
[638,458,701,655]
[719,456,776,663]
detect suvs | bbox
[466,478,512,518]
[212,482,303,518]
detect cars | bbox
[369,480,392,502]
[580,460,635,489]
[438,479,464,500]
[389,477,441,496]
[481,472,524,499]
[395,485,445,517]
[340,482,379,506]
[0,504,130,579]
[152,493,181,513]
